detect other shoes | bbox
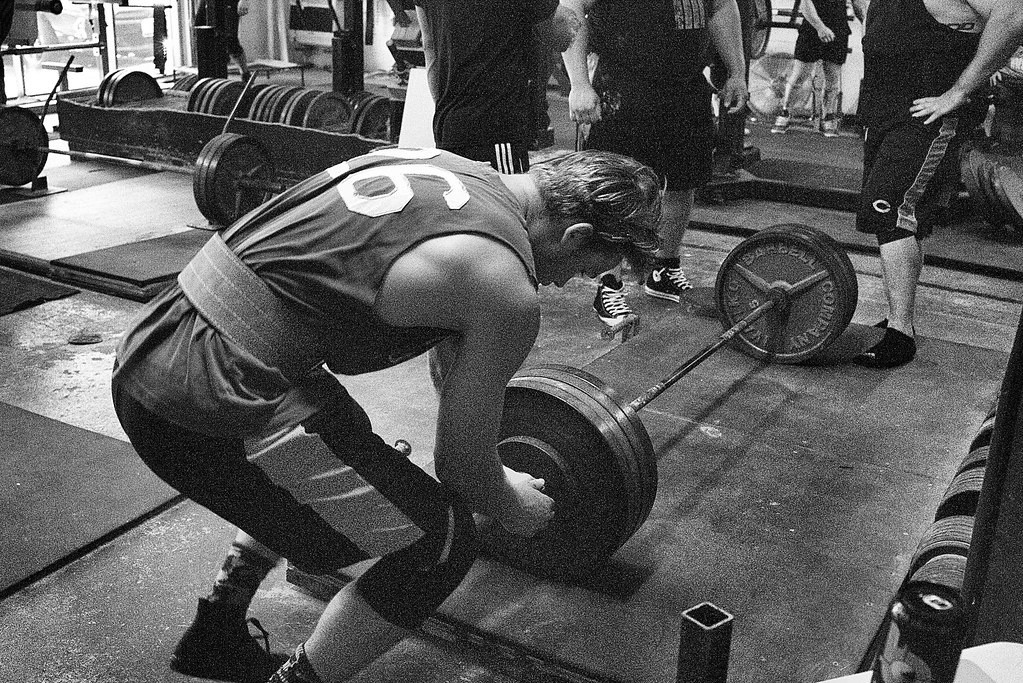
[852,318,917,366]
[170,599,288,682]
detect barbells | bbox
[489,222,860,576]
[0,104,283,229]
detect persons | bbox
[223,0,252,83]
[111,139,666,683]
[558,0,748,327]
[771,0,867,137]
[415,1,580,175]
[852,0,1023,370]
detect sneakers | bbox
[642,256,697,303]
[772,111,793,135]
[819,114,840,139]
[591,272,635,328]
